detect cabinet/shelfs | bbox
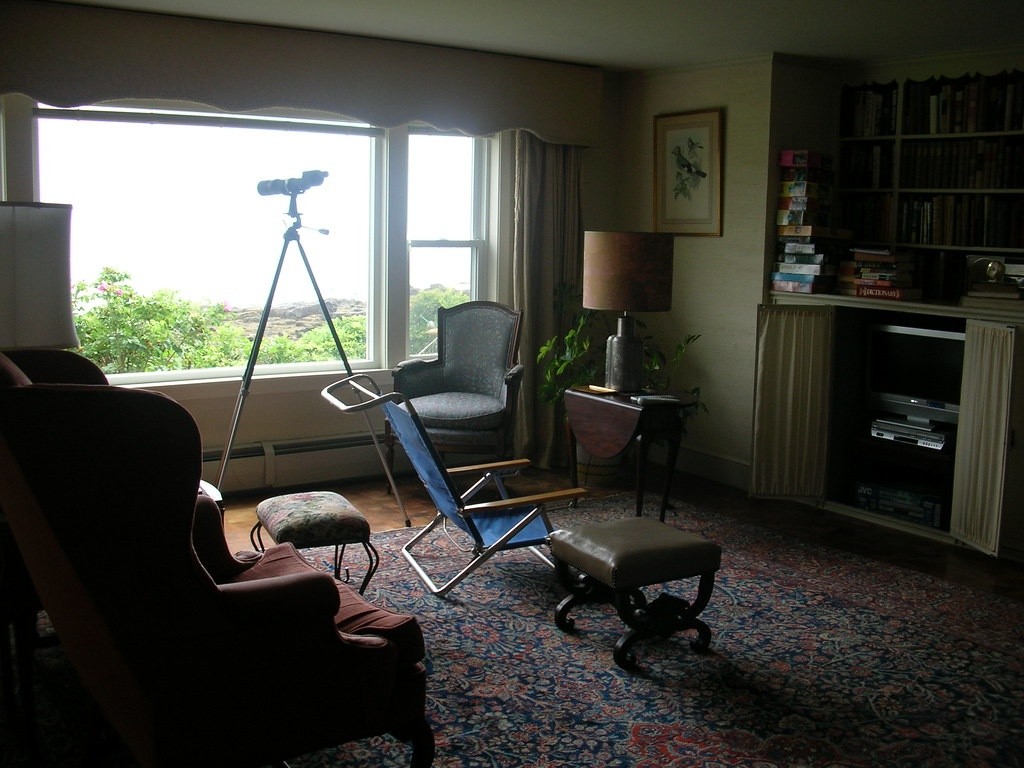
[826,45,1024,288]
[746,289,1024,563]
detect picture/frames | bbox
[652,107,724,237]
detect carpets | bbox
[286,479,1024,768]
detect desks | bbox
[563,389,699,522]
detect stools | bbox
[250,491,380,595]
[549,516,723,670]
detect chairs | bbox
[321,373,586,597]
[385,300,525,495]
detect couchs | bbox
[0,351,435,768]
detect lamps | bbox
[0,201,81,350]
[582,230,673,392]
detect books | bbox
[854,84,1024,247]
[839,250,922,301]
[960,279,1024,311]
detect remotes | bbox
[630,395,682,406]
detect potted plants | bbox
[537,282,710,488]
